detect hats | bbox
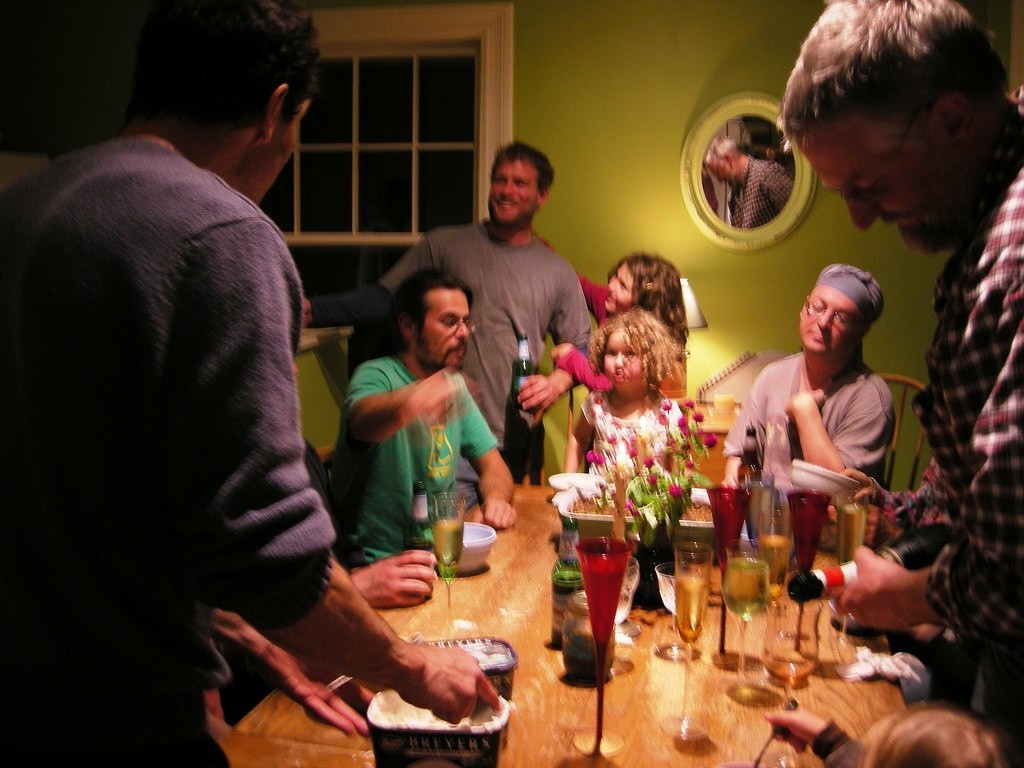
[815,263,883,323]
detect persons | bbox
[707,137,794,230]
[763,701,1009,768]
[561,305,687,484]
[328,267,518,565]
[720,264,897,491]
[481,218,692,470]
[0,0,504,768]
[213,362,438,727]
[777,0,1024,768]
[304,138,594,511]
[839,387,981,706]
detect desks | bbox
[689,405,738,486]
[217,482,908,768]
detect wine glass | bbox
[573,536,637,754]
[700,484,752,664]
[434,491,465,638]
[829,489,870,669]
[780,488,832,666]
[662,542,712,738]
[767,597,821,768]
[653,561,694,655]
[750,509,788,671]
[719,540,771,698]
[609,557,640,675]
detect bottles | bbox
[552,517,585,650]
[513,332,533,400]
[790,521,951,602]
[739,425,762,487]
[405,480,434,553]
[562,589,615,681]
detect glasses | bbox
[435,314,479,335]
[804,299,850,329]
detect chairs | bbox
[876,372,928,492]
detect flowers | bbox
[586,397,719,549]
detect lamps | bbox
[681,278,708,331]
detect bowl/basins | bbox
[548,473,605,494]
[791,460,860,501]
[422,521,497,576]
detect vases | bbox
[633,539,675,613]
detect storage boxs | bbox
[424,637,519,702]
[366,689,512,768]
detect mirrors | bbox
[678,89,819,254]
